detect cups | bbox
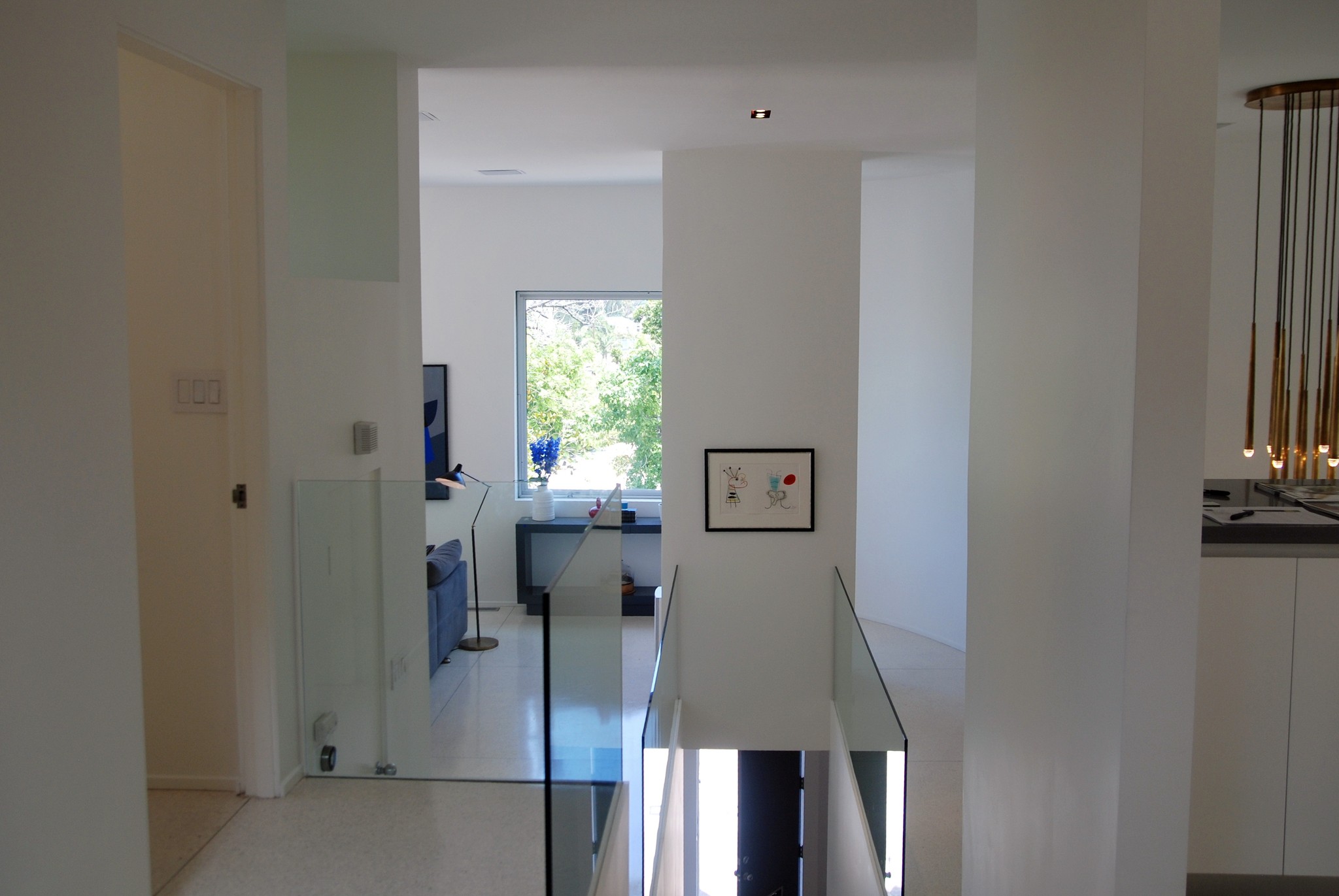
[658,503,662,521]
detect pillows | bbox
[426,539,462,587]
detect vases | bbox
[532,485,555,521]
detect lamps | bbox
[436,463,499,651]
[1244,78,1339,482]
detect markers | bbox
[1230,510,1255,520]
[1204,489,1230,496]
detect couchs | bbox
[426,539,468,680]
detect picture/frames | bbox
[423,364,449,500]
[704,448,815,532]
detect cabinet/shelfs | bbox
[1186,553,1339,896]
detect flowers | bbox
[514,434,561,486]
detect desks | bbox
[515,516,662,616]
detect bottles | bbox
[589,497,610,521]
